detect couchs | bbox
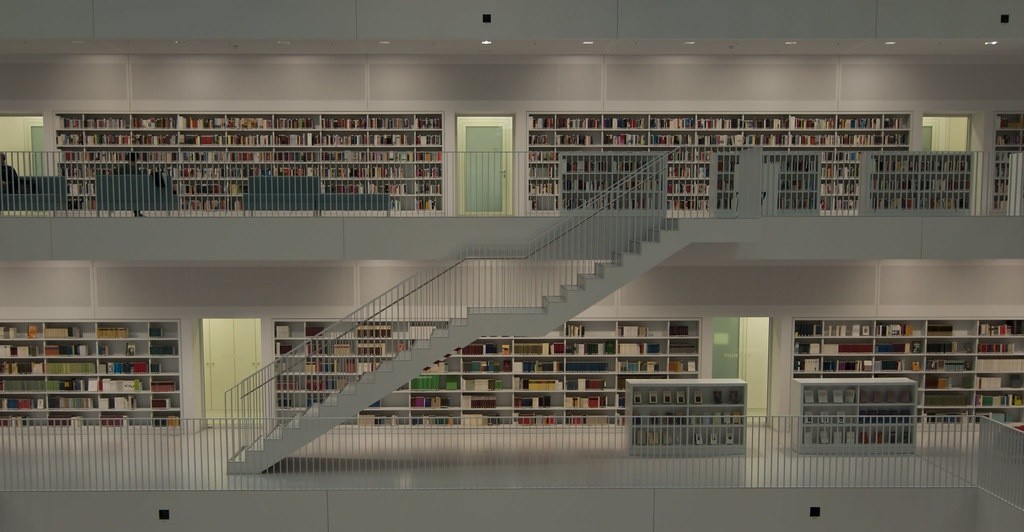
[95,174,178,212]
[241,176,319,212]
[317,193,391,211]
[0,175,68,211]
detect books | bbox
[795,320,1024,444]
[529,115,1024,211]
[276,322,699,427]
[0,323,179,426]
[57,116,441,210]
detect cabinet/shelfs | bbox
[526,111,1022,213]
[0,320,1024,456]
[57,111,445,211]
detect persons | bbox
[121,153,143,217]
[1,154,19,194]
